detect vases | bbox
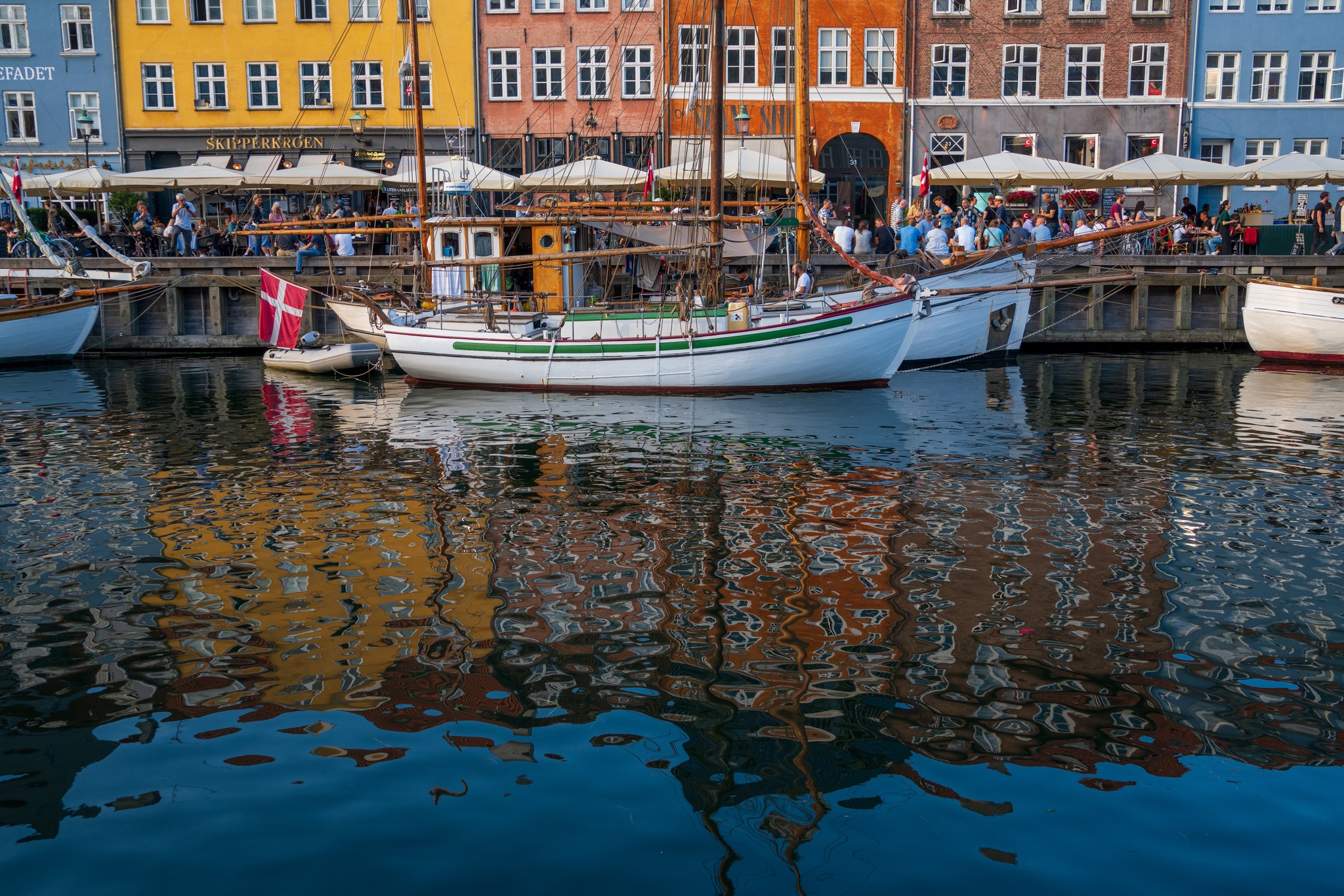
[1069,202,1092,207]
[1005,203,1028,207]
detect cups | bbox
[1241,228,1244,233]
[1197,227,1200,233]
[919,211,922,213]
[1190,227,1196,230]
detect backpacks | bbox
[322,234,334,247]
[340,209,355,227]
[208,249,220,257]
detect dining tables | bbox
[1186,230,1210,255]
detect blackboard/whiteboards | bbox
[1290,243,1302,255]
[1295,193,1308,219]
[287,194,301,214]
[387,195,400,211]
[456,194,470,214]
[809,190,820,207]
[1038,187,1059,214]
[1100,187,1122,216]
[338,194,353,212]
[235,195,248,214]
[1295,233,1304,255]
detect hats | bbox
[993,196,1004,201]
[334,200,345,209]
[313,226,321,229]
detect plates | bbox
[1187,231,1196,233]
[1200,232,1207,233]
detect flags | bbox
[644,153,654,199]
[11,161,22,204]
[262,383,315,445]
[919,154,929,197]
[258,267,308,349]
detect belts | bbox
[279,248,294,251]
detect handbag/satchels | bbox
[1214,216,1220,233]
[1206,236,1214,241]
[1229,226,1235,237]
[132,218,148,231]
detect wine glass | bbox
[906,212,910,218]
[959,210,961,216]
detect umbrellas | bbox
[0,151,826,216]
[913,151,1344,225]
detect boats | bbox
[1241,276,1344,365]
[261,329,381,375]
[1236,360,1344,422]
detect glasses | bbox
[974,200,978,201]
[1035,221,1039,222]
[1182,201,1188,204]
[1082,202,1085,203]
[256,194,261,196]
[276,208,280,210]
[1211,219,1215,221]
[690,198,696,201]
[1061,199,1065,203]
[178,199,186,202]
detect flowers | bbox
[1061,189,1099,206]
[1005,190,1036,205]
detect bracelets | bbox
[1145,220,1146,222]
[189,210,192,213]
[1319,226,1323,227]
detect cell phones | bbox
[144,208,147,213]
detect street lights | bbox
[75,104,94,210]
[734,101,751,149]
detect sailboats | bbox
[223,0,1191,393]
[1,92,162,363]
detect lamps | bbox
[233,163,243,170]
[283,161,294,168]
[232,130,242,143]
[277,130,286,143]
[384,161,394,168]
[337,161,345,165]
[347,109,373,147]
[585,118,598,128]
[102,159,112,171]
[210,130,220,143]
[255,129,264,142]
[299,128,308,141]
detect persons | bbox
[133,200,157,257]
[1134,201,1150,255]
[516,193,531,217]
[1218,200,1239,255]
[595,196,690,249]
[1310,192,1344,255]
[44,193,430,275]
[726,265,755,304]
[783,263,811,298]
[166,192,197,257]
[0,219,26,258]
[754,192,1242,255]
[1111,192,1126,228]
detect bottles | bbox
[1246,203,1249,214]
[1245,203,1246,207]
[1237,208,1244,213]
[1250,203,1252,213]
[933,215,941,219]
[1255,203,1257,213]
[1328,209,1330,214]
[1040,207,1051,219]
[1330,208,1332,214]
[1244,204,1246,213]
[1306,209,1314,218]
[1256,205,1262,213]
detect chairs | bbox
[227,232,250,256]
[1157,227,1260,255]
[109,234,134,256]
[197,232,221,257]
[80,235,103,257]
[156,234,178,257]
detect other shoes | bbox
[243,254,249,256]
[1324,252,1330,255]
[199,254,207,257]
[291,271,302,276]
[289,251,297,257]
[166,246,177,253]
[1188,252,1196,255]
[1205,253,1212,255]
[1179,252,1186,255]
[1312,252,1322,256]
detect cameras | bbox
[182,202,189,209]
